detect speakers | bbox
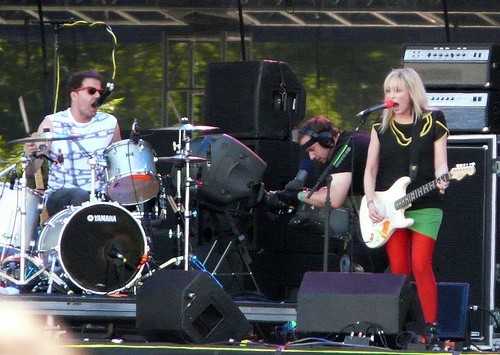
[133,269,252,346]
[168,61,308,208]
[297,145,488,340]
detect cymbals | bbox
[7,131,83,143]
[149,124,217,131]
[158,154,211,166]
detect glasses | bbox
[74,86,105,95]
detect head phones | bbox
[300,128,335,149]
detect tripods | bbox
[0,143,75,298]
[135,130,222,286]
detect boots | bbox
[425,321,443,352]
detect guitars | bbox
[359,162,476,248]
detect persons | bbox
[297,68,451,351]
[24,71,122,226]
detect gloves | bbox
[273,188,304,205]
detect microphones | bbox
[358,99,394,117]
[109,249,124,260]
[94,86,114,107]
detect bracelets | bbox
[368,200,375,207]
[300,192,305,202]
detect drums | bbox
[1,182,42,259]
[37,202,152,296]
[103,139,160,206]
